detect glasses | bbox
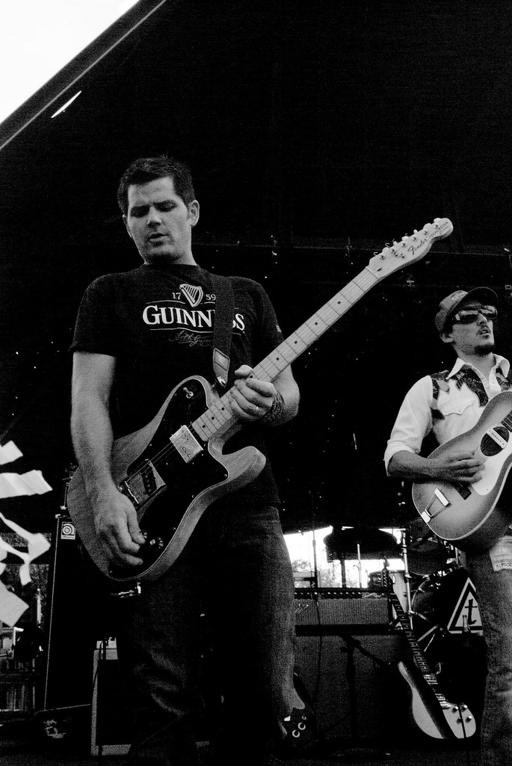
[446,303,498,330]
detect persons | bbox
[382,285,511,766]
[69,158,313,766]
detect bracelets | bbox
[249,390,285,429]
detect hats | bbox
[434,287,499,332]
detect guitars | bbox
[411,391,511,555]
[382,568,477,740]
[67,218,453,589]
